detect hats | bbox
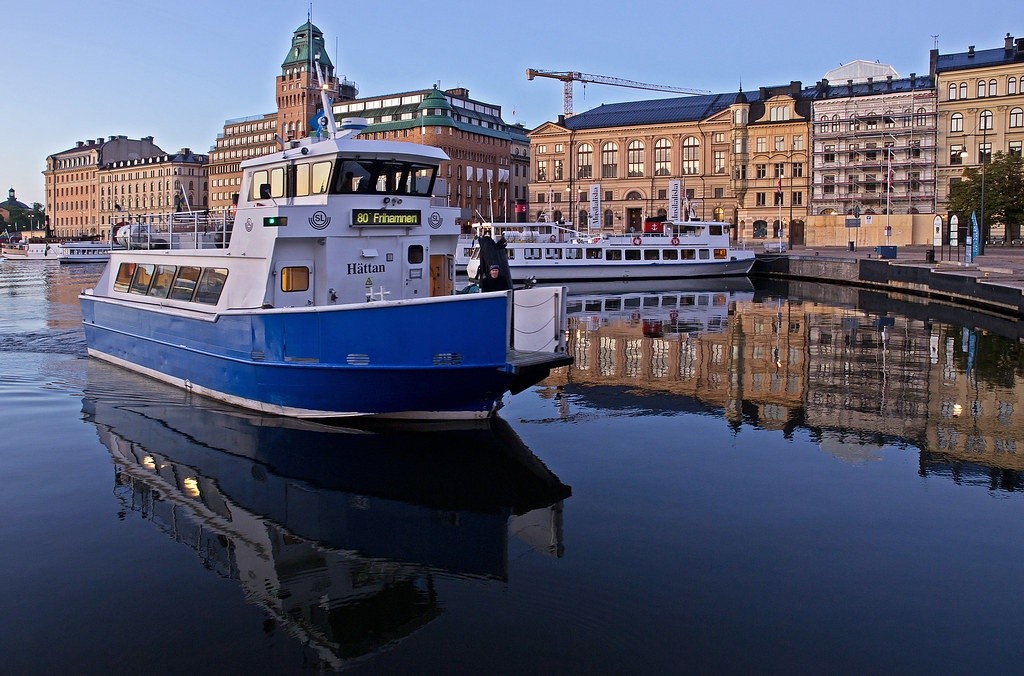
[489,264,499,270]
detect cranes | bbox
[526,67,713,119]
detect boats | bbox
[82,355,574,676]
[513,277,757,322]
[57,240,112,264]
[451,178,757,281]
[77,4,577,423]
[2,243,63,261]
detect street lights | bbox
[28,214,35,240]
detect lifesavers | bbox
[61,241,66,245]
[550,235,556,242]
[671,238,680,245]
[633,237,642,246]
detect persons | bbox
[481,261,510,293]
